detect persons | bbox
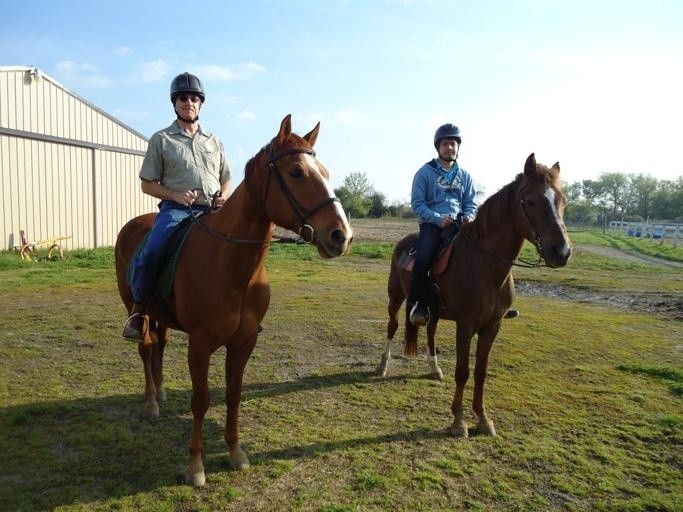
[407,123,520,326]
[121,70,233,343]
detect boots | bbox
[123,303,146,340]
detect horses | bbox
[115,113,354,488]
[375,153,573,438]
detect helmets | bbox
[434,123,461,146]
[170,72,205,103]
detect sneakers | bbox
[412,307,427,326]
[503,310,519,319]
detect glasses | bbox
[180,95,199,102]
